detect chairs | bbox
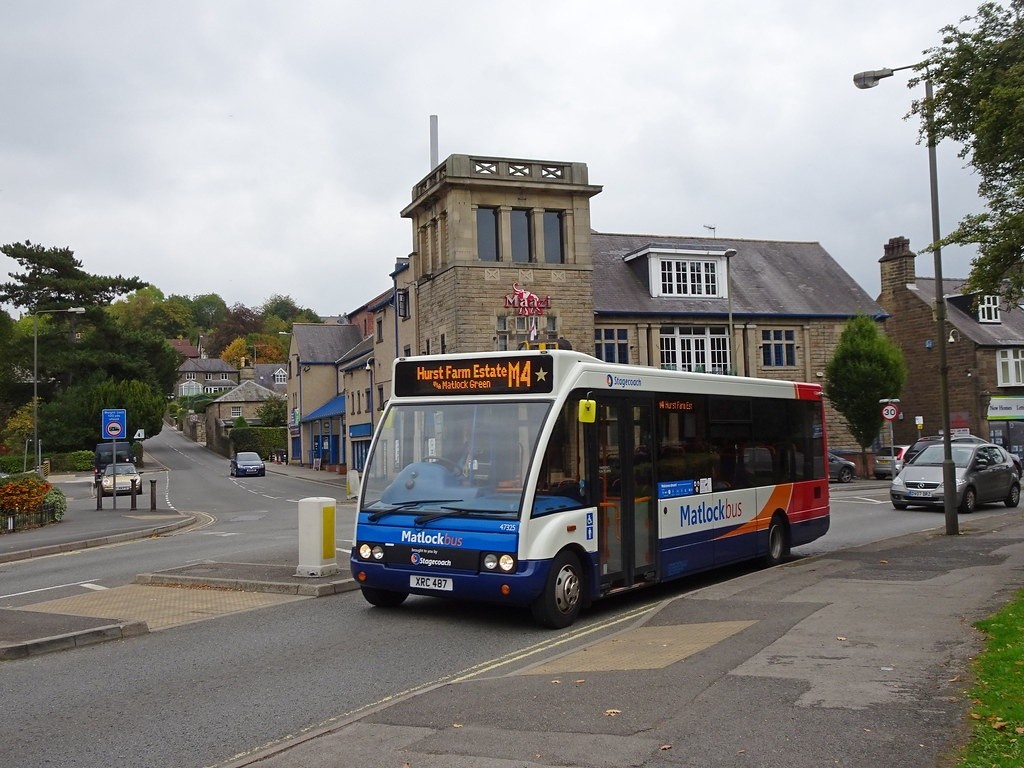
[604,439,800,496]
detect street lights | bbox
[853,61,963,532]
[33,307,86,472]
[365,356,382,478]
[724,248,738,377]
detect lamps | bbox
[366,357,381,372]
[7,512,15,531]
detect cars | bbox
[100,462,144,497]
[827,452,857,484]
[889,441,1020,515]
[230,451,265,477]
[872,444,911,479]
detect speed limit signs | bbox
[882,403,899,422]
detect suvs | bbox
[900,428,1024,486]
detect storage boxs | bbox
[550,469,565,484]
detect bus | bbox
[350,349,831,629]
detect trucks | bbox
[95,441,137,488]
